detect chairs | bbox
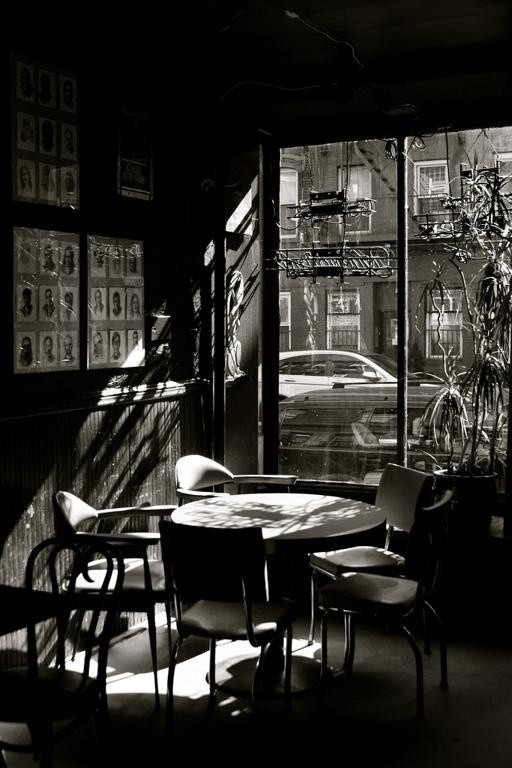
[317,489,454,722]
[350,421,397,481]
[306,461,437,647]
[1,531,124,768]
[48,489,178,715]
[174,454,299,508]
[158,519,299,729]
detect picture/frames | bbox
[116,111,155,202]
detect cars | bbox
[258,349,507,481]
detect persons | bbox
[14,237,144,367]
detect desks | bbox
[0,581,66,640]
[170,492,386,701]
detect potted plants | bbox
[416,149,512,560]
